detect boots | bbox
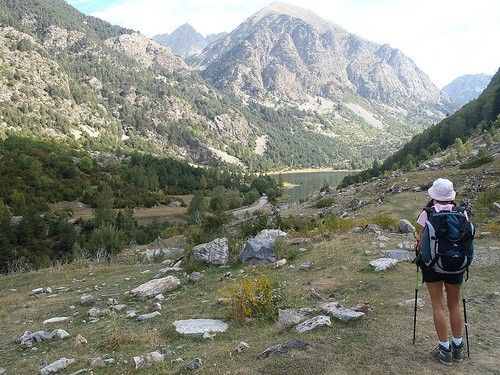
[451,339,465,362]
[432,344,453,365]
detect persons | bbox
[415,177,476,365]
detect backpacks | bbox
[419,201,475,276]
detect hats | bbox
[427,177,457,202]
